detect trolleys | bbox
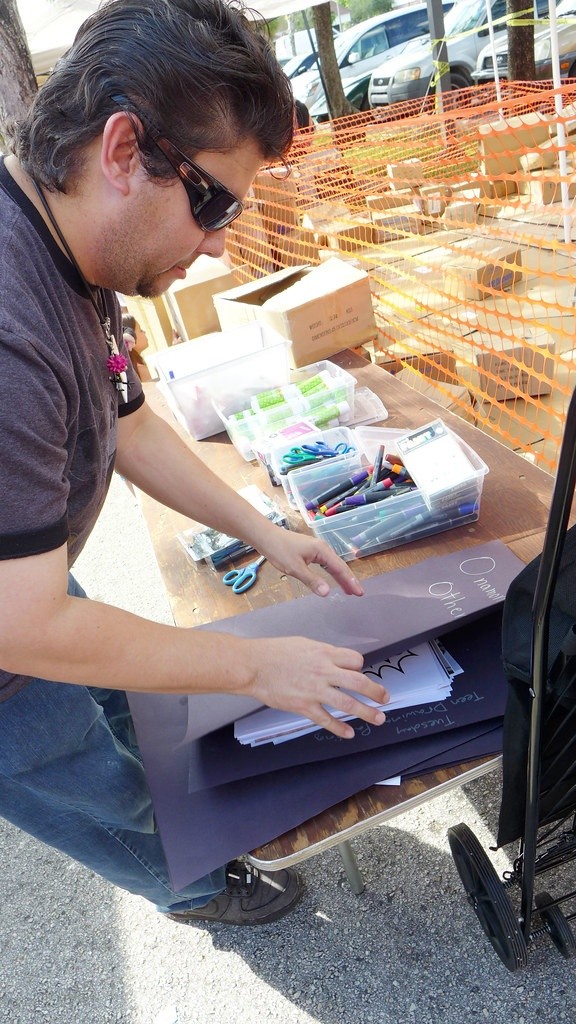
[445,384,574,979]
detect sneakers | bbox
[159,859,305,927]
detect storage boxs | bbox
[117,102,576,561]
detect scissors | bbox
[277,429,355,479]
[223,555,266,596]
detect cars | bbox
[281,1,576,121]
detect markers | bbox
[210,518,289,571]
[305,443,480,558]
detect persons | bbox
[0,0,390,923]
[120,315,152,505]
[263,98,318,169]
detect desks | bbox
[73,334,576,896]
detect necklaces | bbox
[31,178,134,402]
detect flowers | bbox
[108,352,129,375]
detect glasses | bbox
[99,75,244,234]
